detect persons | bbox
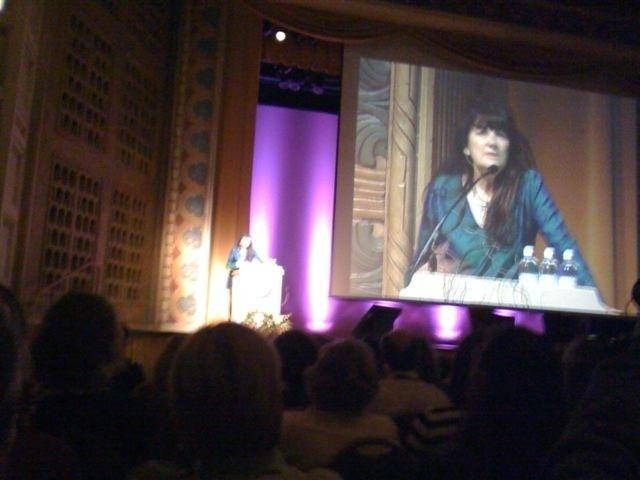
[1,274,640,480]
[400,97,622,314]
[225,233,265,323]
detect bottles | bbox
[516,242,580,307]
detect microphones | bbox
[454,243,484,273]
[403,164,500,285]
